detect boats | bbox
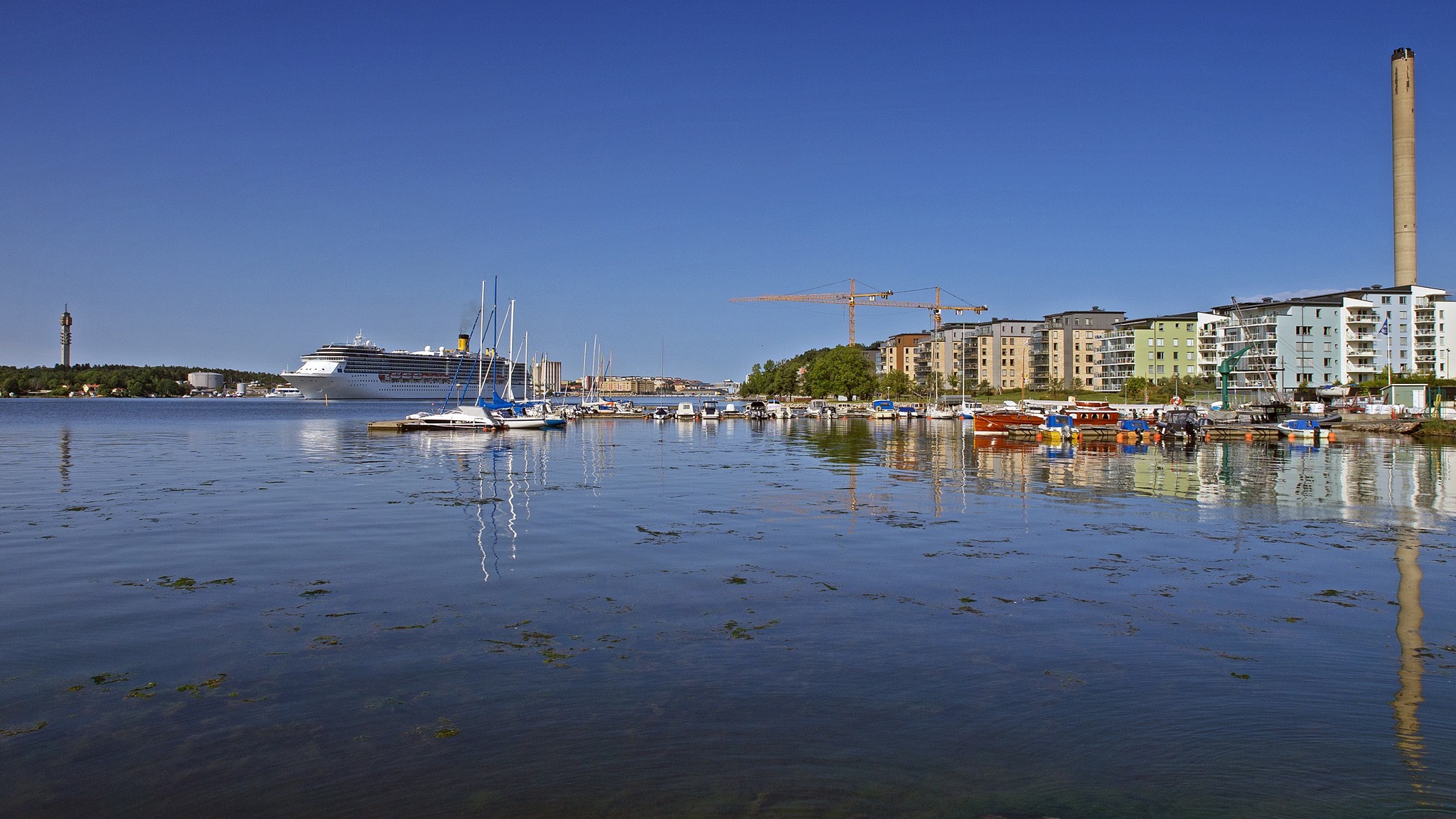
[402,373,412,379]
[388,372,401,378]
[266,383,304,398]
[207,390,245,399]
[440,374,450,380]
[379,376,392,381]
[497,376,506,380]
[481,376,490,380]
[490,376,498,380]
[412,373,422,379]
[422,374,432,379]
[651,340,1254,444]
[1278,419,1336,443]
[431,374,441,379]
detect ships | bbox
[280,328,532,403]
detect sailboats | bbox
[395,278,646,429]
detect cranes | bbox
[728,277,891,346]
[761,287,990,328]
